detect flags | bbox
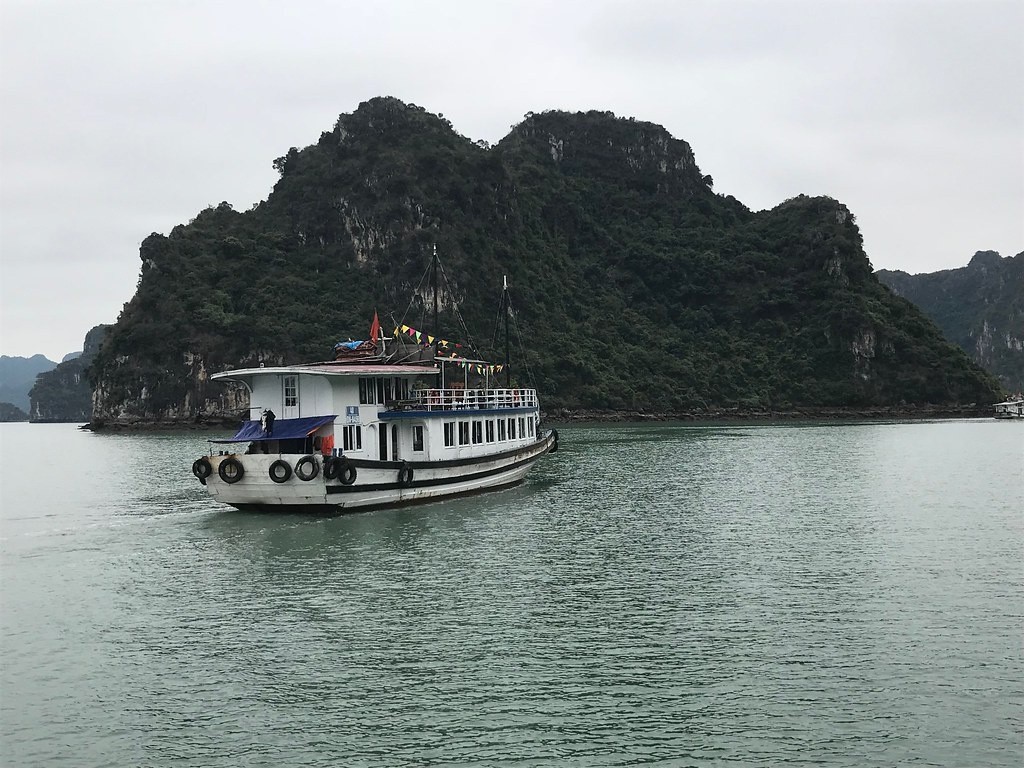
[370,313,379,340]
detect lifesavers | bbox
[192,459,212,486]
[293,455,320,481]
[218,457,245,484]
[323,456,357,485]
[397,464,414,488]
[268,459,293,484]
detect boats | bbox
[196,241,557,515]
[992,390,1024,420]
